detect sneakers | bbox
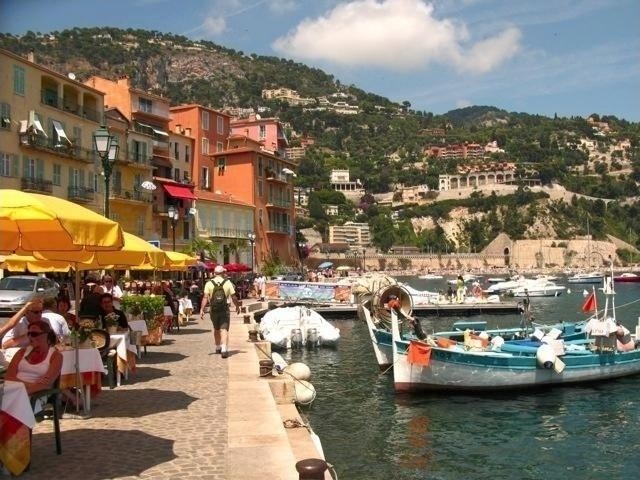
[216,345,228,357]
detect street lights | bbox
[247,231,256,270]
[167,205,180,252]
[93,125,121,219]
[296,241,307,275]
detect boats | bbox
[363,305,619,374]
[339,267,567,304]
[391,262,640,394]
[258,306,341,352]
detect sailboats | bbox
[567,217,604,285]
[613,228,638,282]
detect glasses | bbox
[26,332,45,337]
[31,310,42,314]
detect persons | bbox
[614,331,637,352]
[444,273,482,304]
[252,269,270,300]
[5,321,62,411]
[199,265,241,359]
[0,271,125,348]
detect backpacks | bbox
[210,279,228,312]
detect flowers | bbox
[104,312,119,323]
[80,318,93,332]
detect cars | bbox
[0,276,62,317]
[271,273,305,281]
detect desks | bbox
[100,326,131,386]
[54,339,104,416]
[2,376,36,477]
[130,318,147,361]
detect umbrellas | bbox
[318,261,334,268]
[0,185,252,285]
[336,264,354,270]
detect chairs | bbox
[82,327,117,391]
[31,364,61,456]
[168,300,180,331]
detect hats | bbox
[214,265,227,272]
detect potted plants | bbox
[119,294,165,326]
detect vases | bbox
[105,324,120,334]
